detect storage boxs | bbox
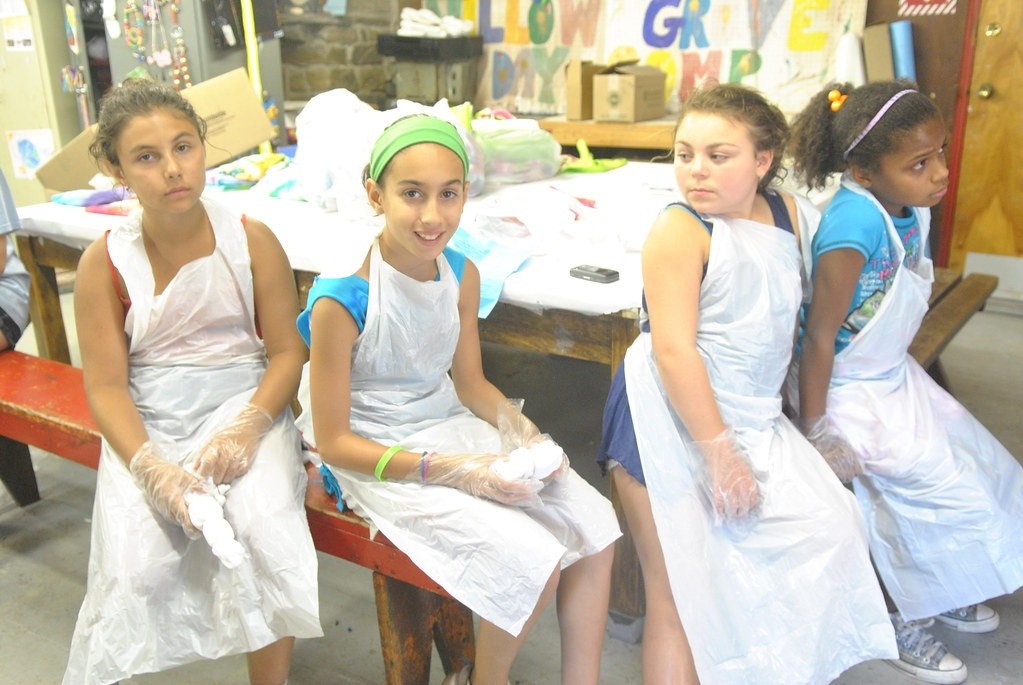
[37,67,276,193]
[563,53,608,120]
[591,59,667,123]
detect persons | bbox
[294,103,626,685]
[785,77,1023,685]
[594,81,898,685]
[0,167,36,356]
[62,77,324,685]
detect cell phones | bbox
[570,263,620,282]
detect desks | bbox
[541,111,679,161]
[15,143,847,626]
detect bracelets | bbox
[420,449,438,487]
[373,445,404,484]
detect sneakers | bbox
[935,604,1001,634]
[882,612,968,685]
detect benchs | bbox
[906,267,1001,393]
[0,349,474,685]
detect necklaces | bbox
[112,0,192,91]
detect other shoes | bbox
[442,662,514,685]
